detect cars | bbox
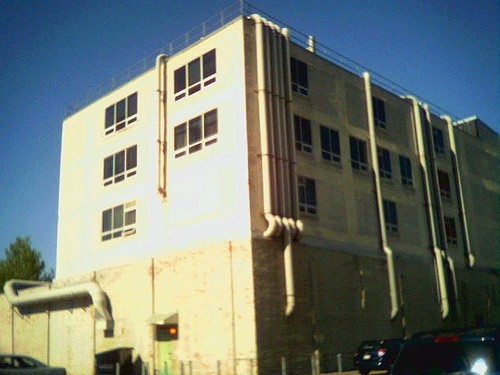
[354,338,399,375]
[1,354,66,375]
[388,329,500,375]
